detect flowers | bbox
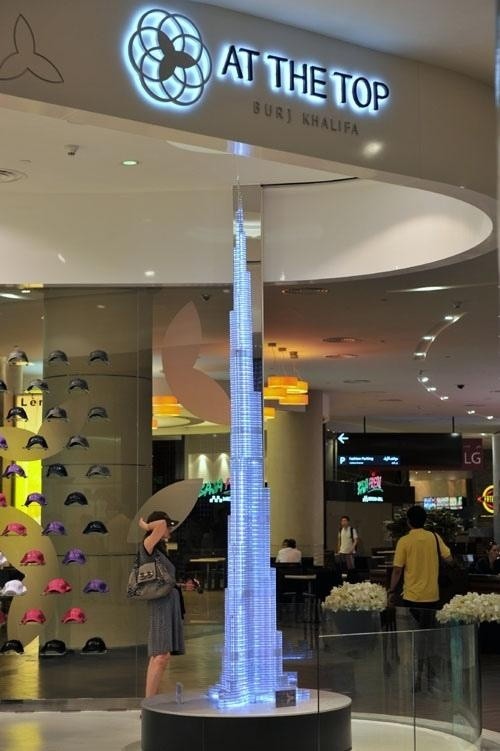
[378,505,464,550]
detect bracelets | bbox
[389,589,395,595]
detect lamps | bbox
[262,341,310,421]
[152,378,181,429]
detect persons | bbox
[475,542,499,574]
[136,512,200,697]
[338,516,358,570]
[388,505,453,691]
[282,539,287,547]
[275,539,302,563]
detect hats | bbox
[146,511,180,529]
[0,347,110,658]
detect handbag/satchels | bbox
[430,534,460,589]
[125,544,175,604]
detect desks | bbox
[188,557,226,594]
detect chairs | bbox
[267,545,500,677]
[208,568,226,591]
[168,549,196,583]
